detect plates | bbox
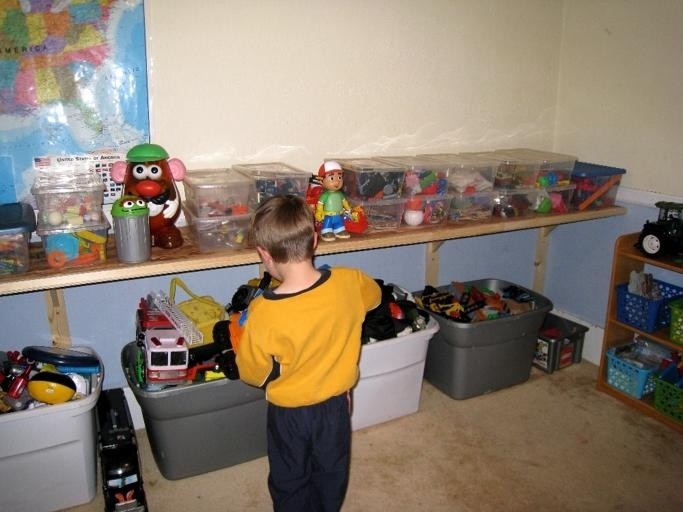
[585,223,683,428]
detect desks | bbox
[0,197,627,357]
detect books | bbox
[533,312,589,378]
[348,281,439,433]
[119,338,275,483]
[0,340,106,510]
[410,274,556,401]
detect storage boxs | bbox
[533,312,589,378]
[0,340,106,510]
[119,338,275,483]
[0,143,629,277]
[348,281,439,433]
[410,274,556,401]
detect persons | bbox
[233,193,395,512]
[315,160,352,244]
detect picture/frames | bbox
[0,197,627,357]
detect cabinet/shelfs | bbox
[585,223,683,428]
